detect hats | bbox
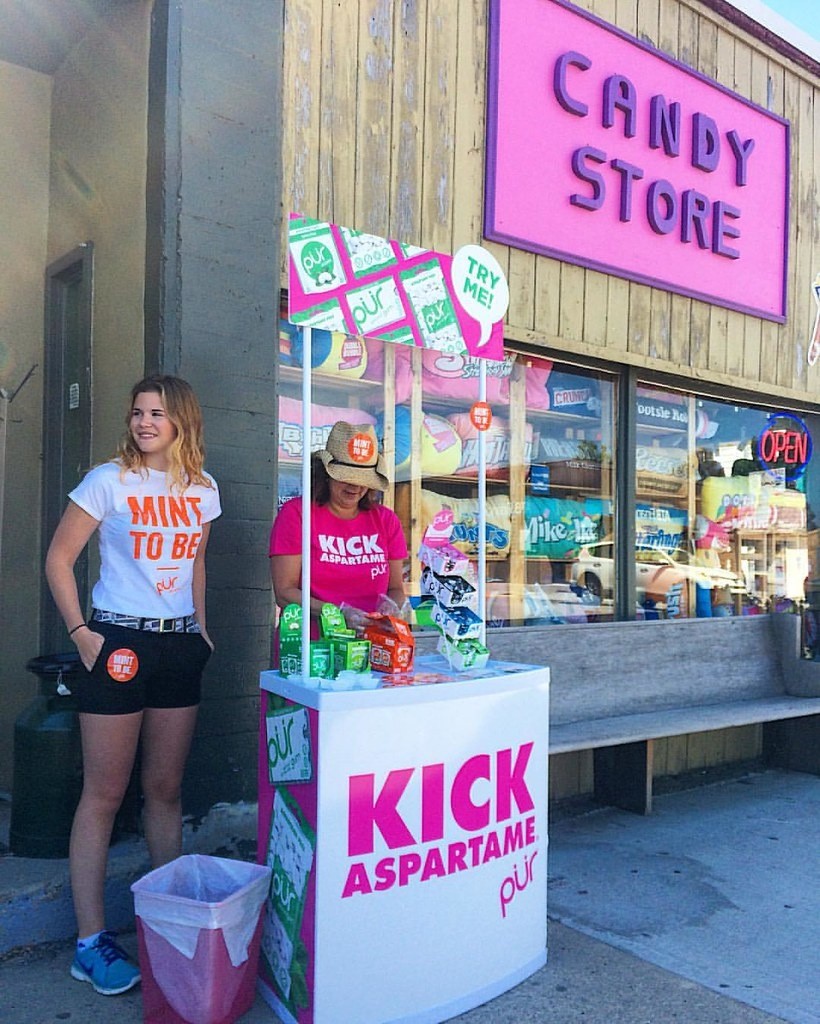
[315,421,390,492]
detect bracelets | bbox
[68,624,86,636]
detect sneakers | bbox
[70,931,140,996]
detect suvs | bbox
[566,539,749,611]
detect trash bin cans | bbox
[128,852,272,1023]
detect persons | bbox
[45,376,223,995]
[269,421,410,669]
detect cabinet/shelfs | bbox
[282,344,696,621]
[724,531,809,617]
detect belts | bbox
[92,610,204,633]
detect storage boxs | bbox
[277,510,490,681]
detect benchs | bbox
[422,613,820,818]
